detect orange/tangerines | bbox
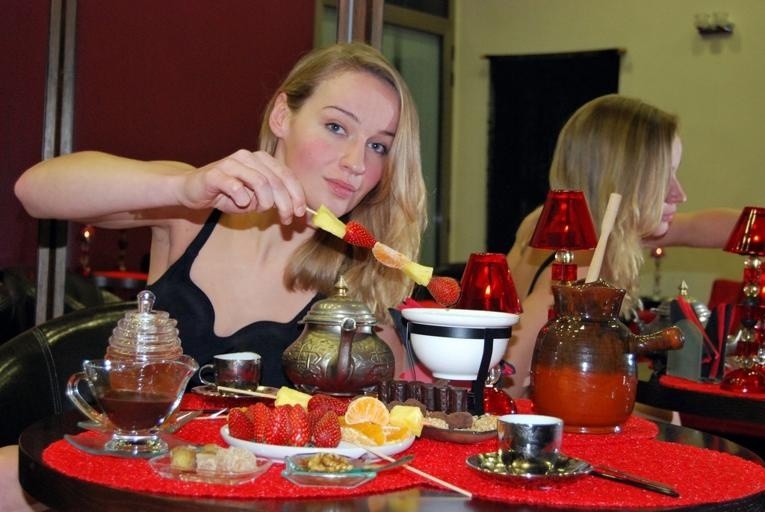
[345,396,390,425]
[372,241,408,269]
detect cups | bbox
[66,354,199,453]
[402,307,520,382]
[494,414,563,475]
[200,351,261,395]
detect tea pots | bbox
[281,275,396,398]
[632,281,710,383]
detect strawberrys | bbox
[228,394,347,448]
[426,276,462,308]
[344,220,377,248]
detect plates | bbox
[465,451,593,484]
[150,453,272,485]
[191,385,279,404]
[220,423,416,463]
[280,451,377,489]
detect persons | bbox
[500,91,744,400]
[11,40,432,396]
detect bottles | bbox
[530,285,685,432]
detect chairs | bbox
[400,262,466,329]
[0,300,141,445]
